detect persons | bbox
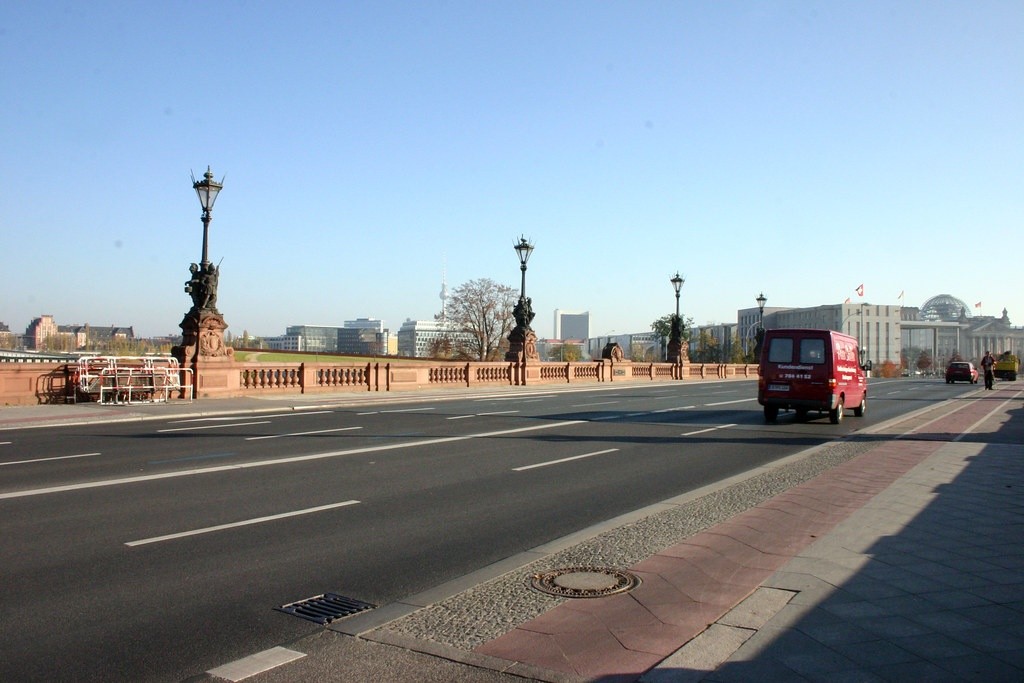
[185,262,220,308]
[513,297,535,327]
[981,351,995,390]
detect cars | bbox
[945,362,979,384]
[902,369,912,377]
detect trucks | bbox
[990,353,1021,382]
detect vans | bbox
[757,330,872,426]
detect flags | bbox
[975,302,981,308]
[856,284,863,296]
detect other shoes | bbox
[988,387,993,390]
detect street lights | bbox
[505,235,541,361]
[753,292,765,365]
[746,320,761,358]
[666,272,691,365]
[169,163,237,364]
[860,303,871,364]
